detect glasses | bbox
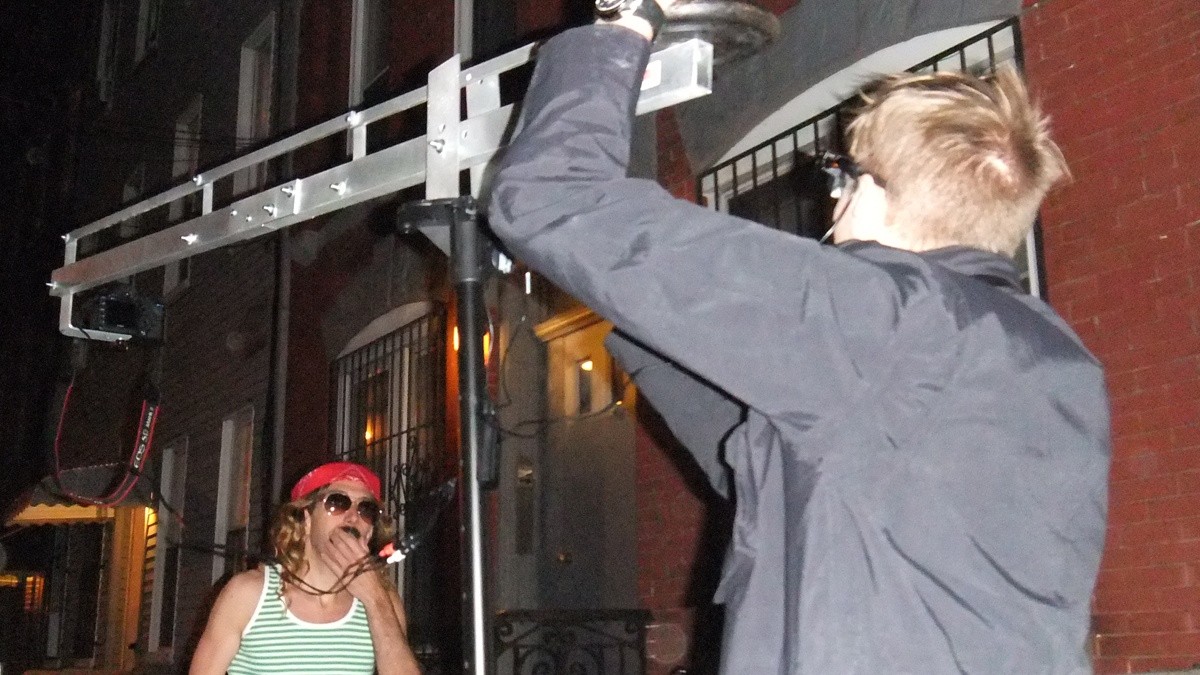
[315,491,380,525]
[819,149,893,199]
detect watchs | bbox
[591,0,665,41]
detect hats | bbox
[290,463,381,503]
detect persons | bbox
[193,461,422,675]
[486,0,1112,674]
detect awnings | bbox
[3,462,152,525]
